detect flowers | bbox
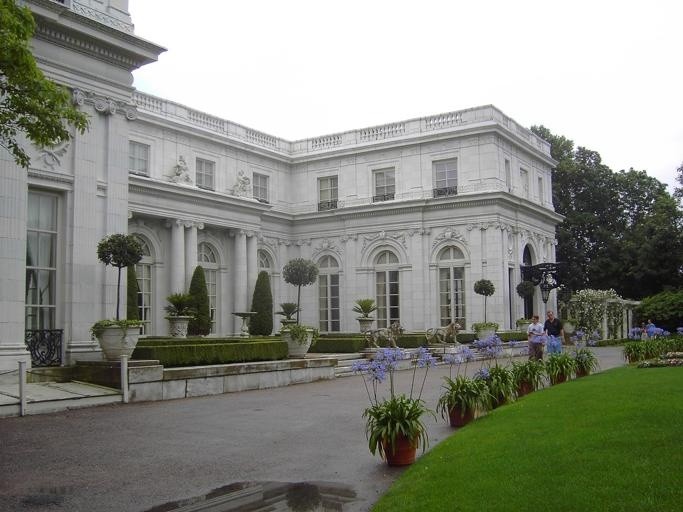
[625,328,683,364]
[510,337,544,380]
[356,343,435,431]
[572,329,601,365]
[478,335,510,389]
[439,341,490,406]
[545,334,574,371]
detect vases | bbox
[447,403,474,429]
[574,366,592,378]
[518,381,535,397]
[489,392,509,409]
[381,433,416,466]
[550,372,567,385]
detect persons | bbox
[525,315,544,363]
[542,310,565,354]
[645,318,656,340]
[639,322,647,340]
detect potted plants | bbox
[93,234,141,360]
[282,258,317,358]
[165,292,194,337]
[471,323,498,343]
[560,320,579,334]
[352,298,377,333]
[518,319,534,333]
